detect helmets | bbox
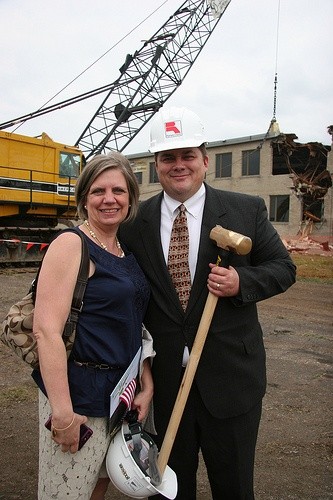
[105,417,178,500]
[148,106,208,155]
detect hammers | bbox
[156,226,252,481]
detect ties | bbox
[167,205,192,314]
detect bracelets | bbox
[51,413,74,437]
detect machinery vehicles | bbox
[0,131,87,269]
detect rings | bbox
[217,283,221,290]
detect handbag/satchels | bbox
[0,226,91,374]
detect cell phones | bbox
[45,418,93,450]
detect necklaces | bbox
[83,220,124,259]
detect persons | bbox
[32,152,154,500]
[116,106,297,500]
